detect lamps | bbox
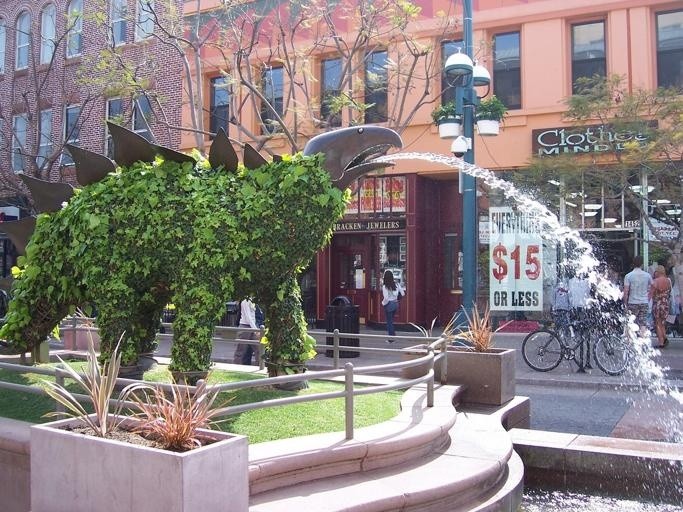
[579,203,618,223]
[630,184,682,215]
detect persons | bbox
[381,270,407,343]
[234,293,260,365]
[551,254,682,350]
[301,268,316,311]
[244,302,266,365]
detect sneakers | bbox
[657,339,669,348]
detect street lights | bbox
[440,46,492,347]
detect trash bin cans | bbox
[324,297,360,358]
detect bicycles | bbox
[521,301,630,376]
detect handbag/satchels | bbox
[669,301,676,315]
[398,292,403,300]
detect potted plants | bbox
[20,305,251,512]
[474,93,510,136]
[400,297,516,406]
[432,99,462,139]
